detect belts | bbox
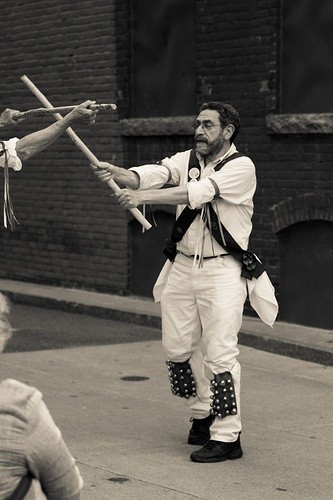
[180,252,229,260]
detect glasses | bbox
[191,121,227,130]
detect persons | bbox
[91,101,258,464]
[0,100,98,172]
[0,291,84,500]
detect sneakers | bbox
[191,434,243,462]
[188,414,217,444]
